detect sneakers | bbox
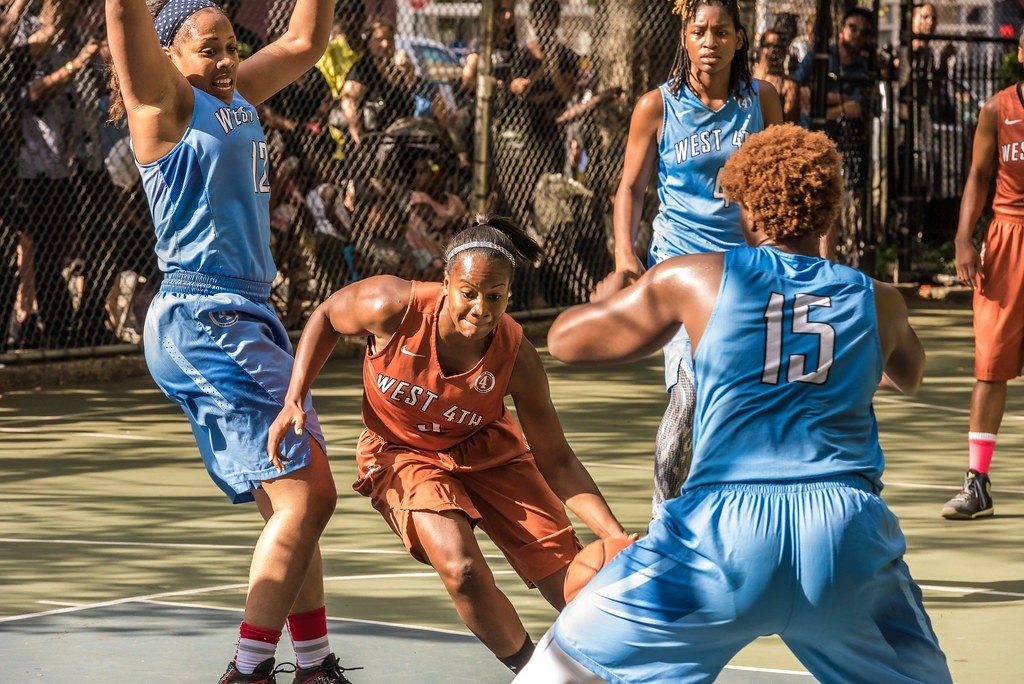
[291,653,354,684]
[217,658,275,684]
[941,468,995,518]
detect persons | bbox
[1,1,1024,354]
[612,0,787,534]
[266,213,640,677]
[103,0,353,683]
[509,121,959,684]
[941,27,1024,521]
